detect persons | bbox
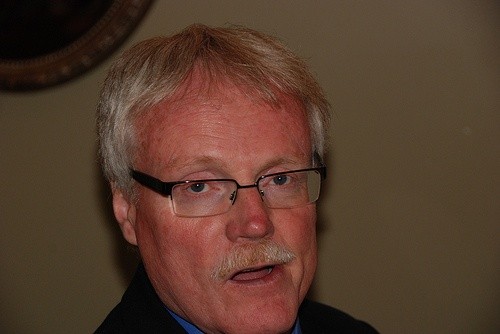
[89,23,379,334]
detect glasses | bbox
[127,151,327,217]
